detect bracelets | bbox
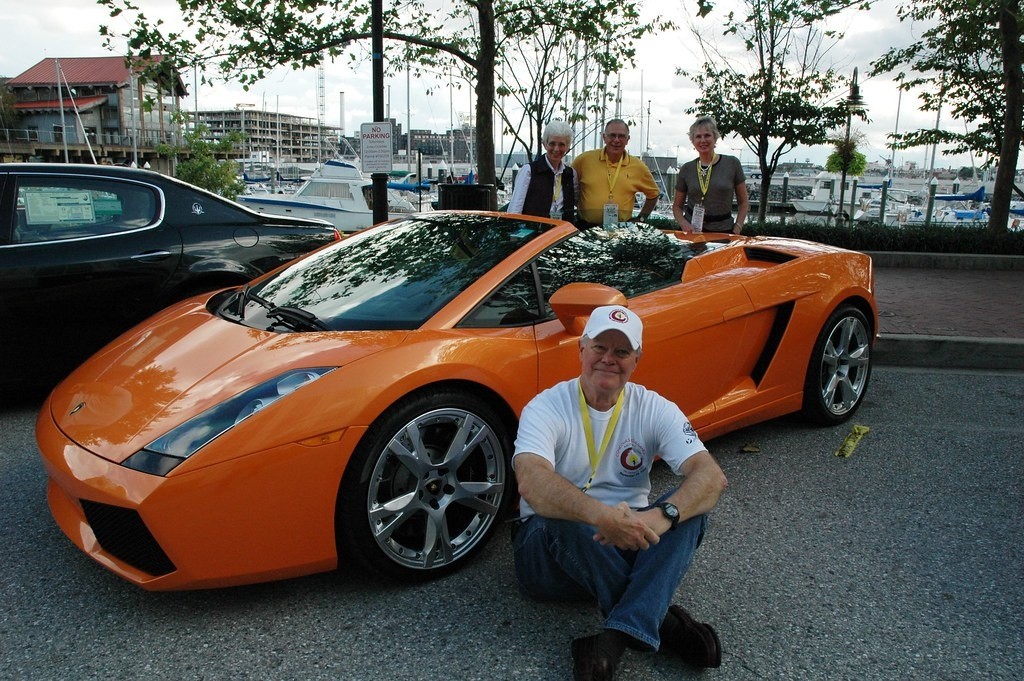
[734,223,742,231]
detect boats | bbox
[787,170,1024,237]
[236,156,418,235]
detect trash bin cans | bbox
[437,182,498,212]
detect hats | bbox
[580,305,645,351]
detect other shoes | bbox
[571,633,623,681]
[658,604,722,667]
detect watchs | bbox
[655,502,680,530]
[638,216,645,222]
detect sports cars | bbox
[34,208,878,595]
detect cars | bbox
[0,161,345,400]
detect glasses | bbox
[605,131,627,138]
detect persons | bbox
[571,119,660,222]
[506,120,579,229]
[673,117,749,235]
[511,306,727,681]
